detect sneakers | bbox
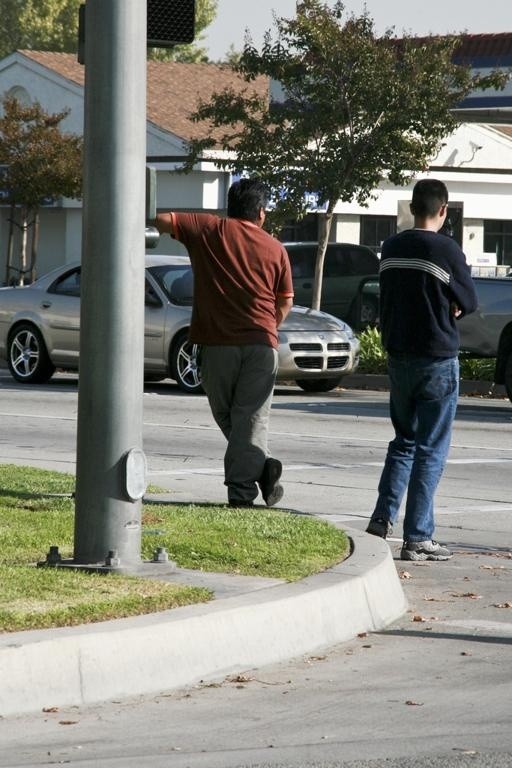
[365,518,390,540]
[258,457,283,506]
[400,540,454,561]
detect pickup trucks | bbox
[452,274,512,401]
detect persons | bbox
[364,177,478,566]
[154,177,295,510]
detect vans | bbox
[272,241,385,330]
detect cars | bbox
[0,251,363,393]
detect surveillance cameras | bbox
[435,142,447,147]
[469,140,482,150]
